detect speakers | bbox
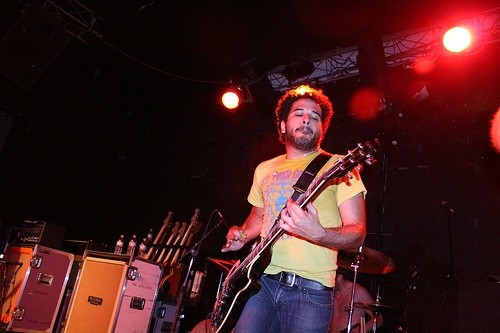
[0,3,74,91]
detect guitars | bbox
[140,209,203,299]
[208,134,384,333]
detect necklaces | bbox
[304,145,321,156]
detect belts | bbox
[263,271,333,292]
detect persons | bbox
[221,84,368,333]
[329,267,384,333]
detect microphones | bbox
[217,210,231,230]
[441,199,456,215]
[334,137,380,177]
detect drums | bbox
[188,319,226,333]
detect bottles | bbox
[147,228,153,241]
[138,237,147,258]
[114,235,124,254]
[126,235,137,256]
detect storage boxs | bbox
[0,242,163,333]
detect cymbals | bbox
[336,245,396,275]
[348,302,402,315]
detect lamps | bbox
[217,85,245,109]
[442,24,475,53]
